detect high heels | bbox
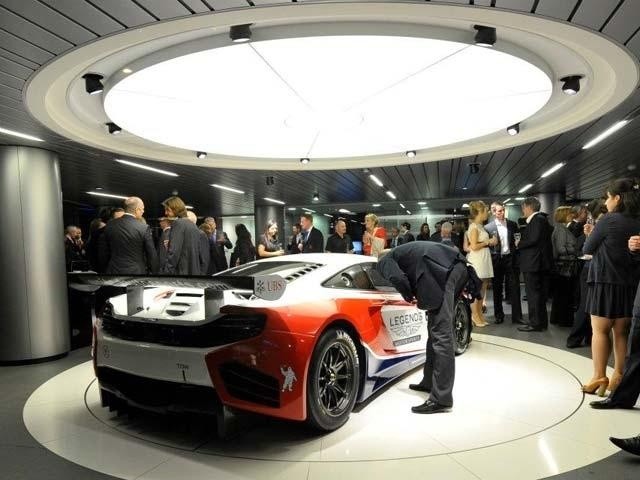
[582,371,624,397]
[472,315,490,327]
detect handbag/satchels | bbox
[555,249,584,279]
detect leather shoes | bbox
[590,396,633,409]
[495,317,534,327]
[609,435,640,455]
[409,383,451,413]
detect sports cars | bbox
[86,250,473,434]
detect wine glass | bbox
[492,231,498,249]
[515,234,521,249]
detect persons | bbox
[230,214,323,266]
[374,239,470,413]
[363,214,387,257]
[579,179,639,396]
[326,220,354,254]
[462,196,609,347]
[590,236,640,408]
[65,196,232,275]
[608,434,640,459]
[386,220,466,248]
